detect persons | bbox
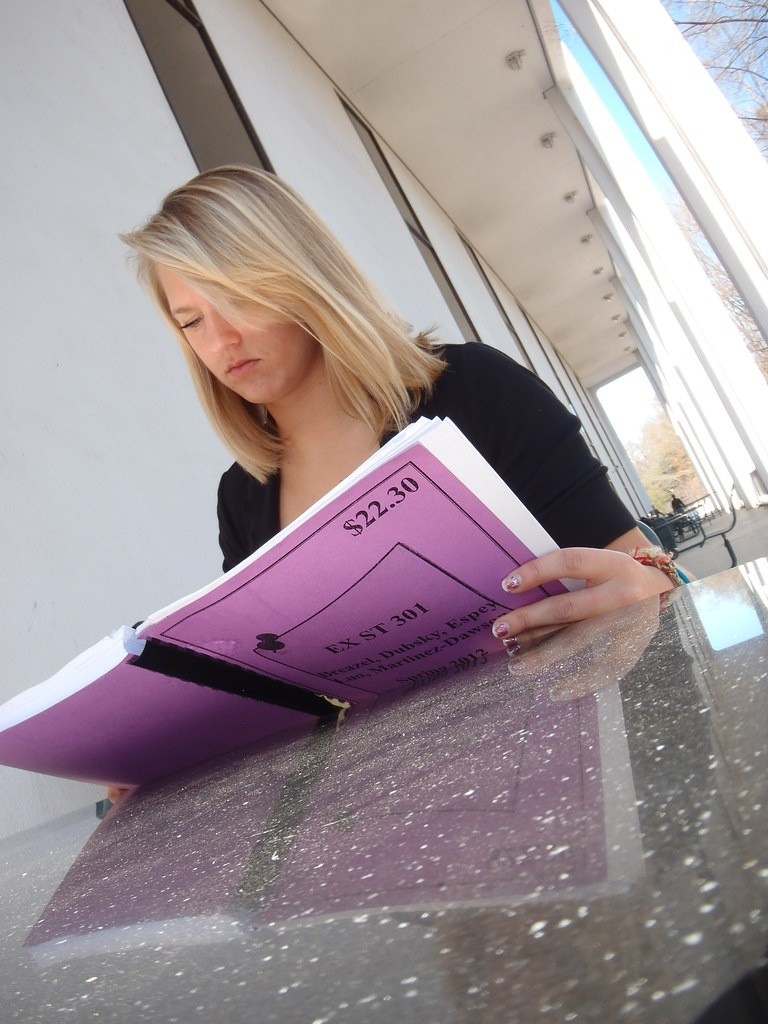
[106,165,675,801]
[651,505,665,518]
[671,495,687,515]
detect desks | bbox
[0,556,768,1024]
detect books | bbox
[1,416,581,792]
[23,636,644,970]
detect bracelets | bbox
[629,546,690,587]
